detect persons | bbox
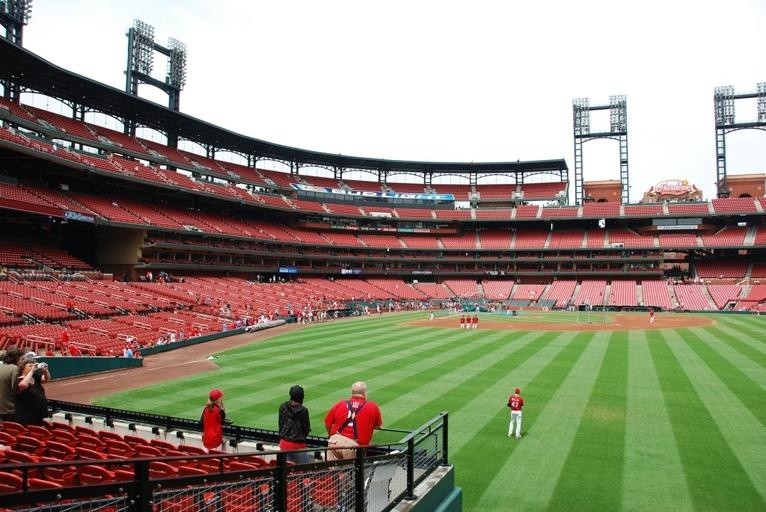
[0,349,50,421]
[200,390,226,450]
[278,385,311,463]
[324,382,383,461]
[507,388,524,438]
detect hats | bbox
[209,389,224,401]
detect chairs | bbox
[1,420,346,512]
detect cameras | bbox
[38,364,44,368]
